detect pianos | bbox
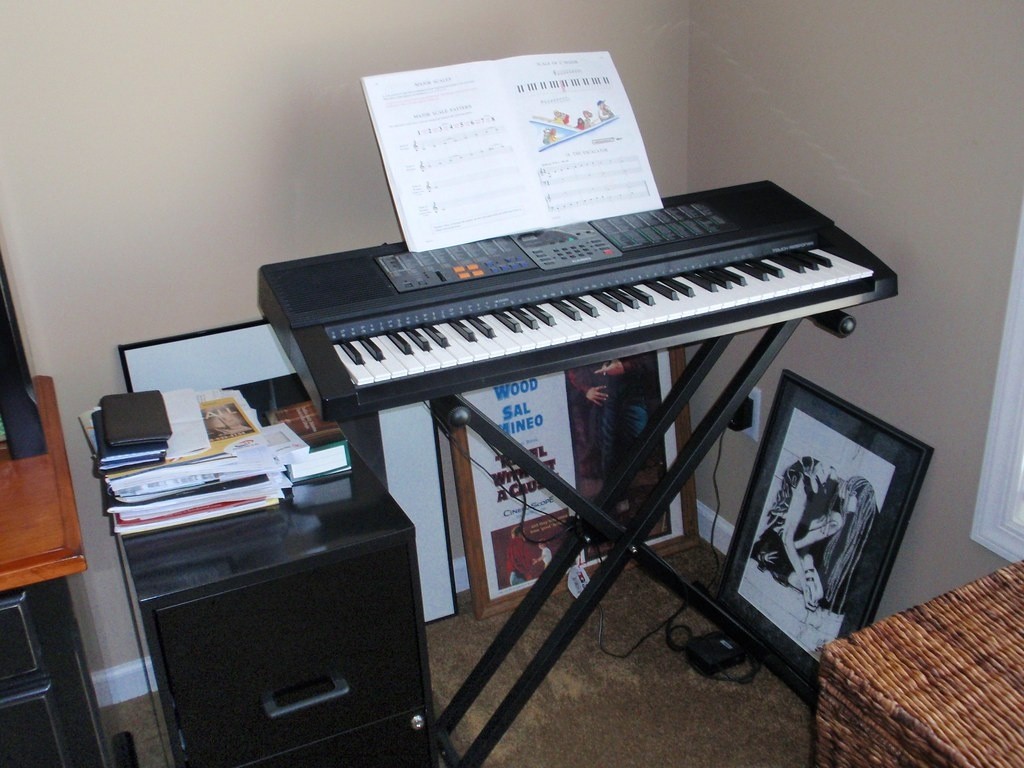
[256,177,898,423]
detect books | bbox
[360,51,666,254]
[74,389,311,538]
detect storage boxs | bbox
[0,375,88,594]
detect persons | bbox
[565,350,668,515]
[508,525,554,589]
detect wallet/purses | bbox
[100,389,173,447]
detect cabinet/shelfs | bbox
[118,442,442,768]
[0,575,112,768]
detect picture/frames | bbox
[717,369,937,701]
[448,346,700,627]
[119,319,462,627]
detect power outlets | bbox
[741,386,763,443]
